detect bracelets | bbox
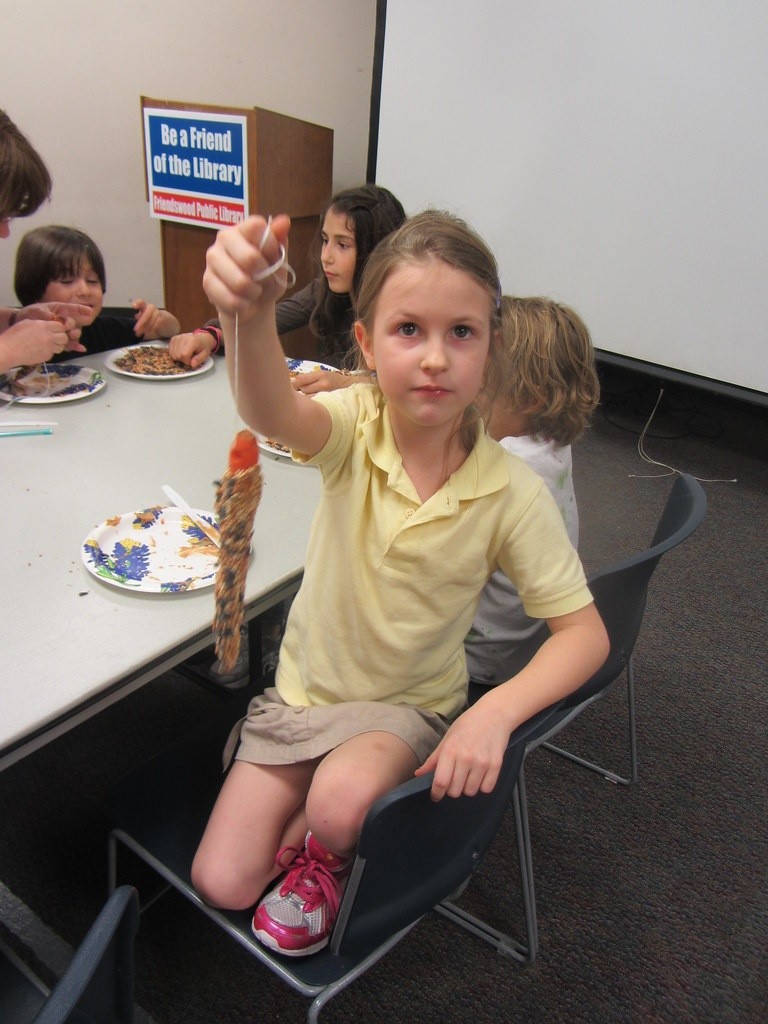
[8,308,21,326]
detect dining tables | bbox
[0,338,377,873]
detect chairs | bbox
[0,885,140,1024]
[438,473,708,954]
[106,696,537,1024]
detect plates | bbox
[285,359,342,384]
[0,363,106,405]
[246,428,293,458]
[80,506,252,592]
[105,344,214,381]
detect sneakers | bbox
[252,830,349,957]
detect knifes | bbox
[0,422,61,426]
[162,485,221,548]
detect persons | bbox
[0,109,92,375]
[463,297,600,709]
[14,226,180,363]
[192,213,610,955]
[171,185,407,398]
